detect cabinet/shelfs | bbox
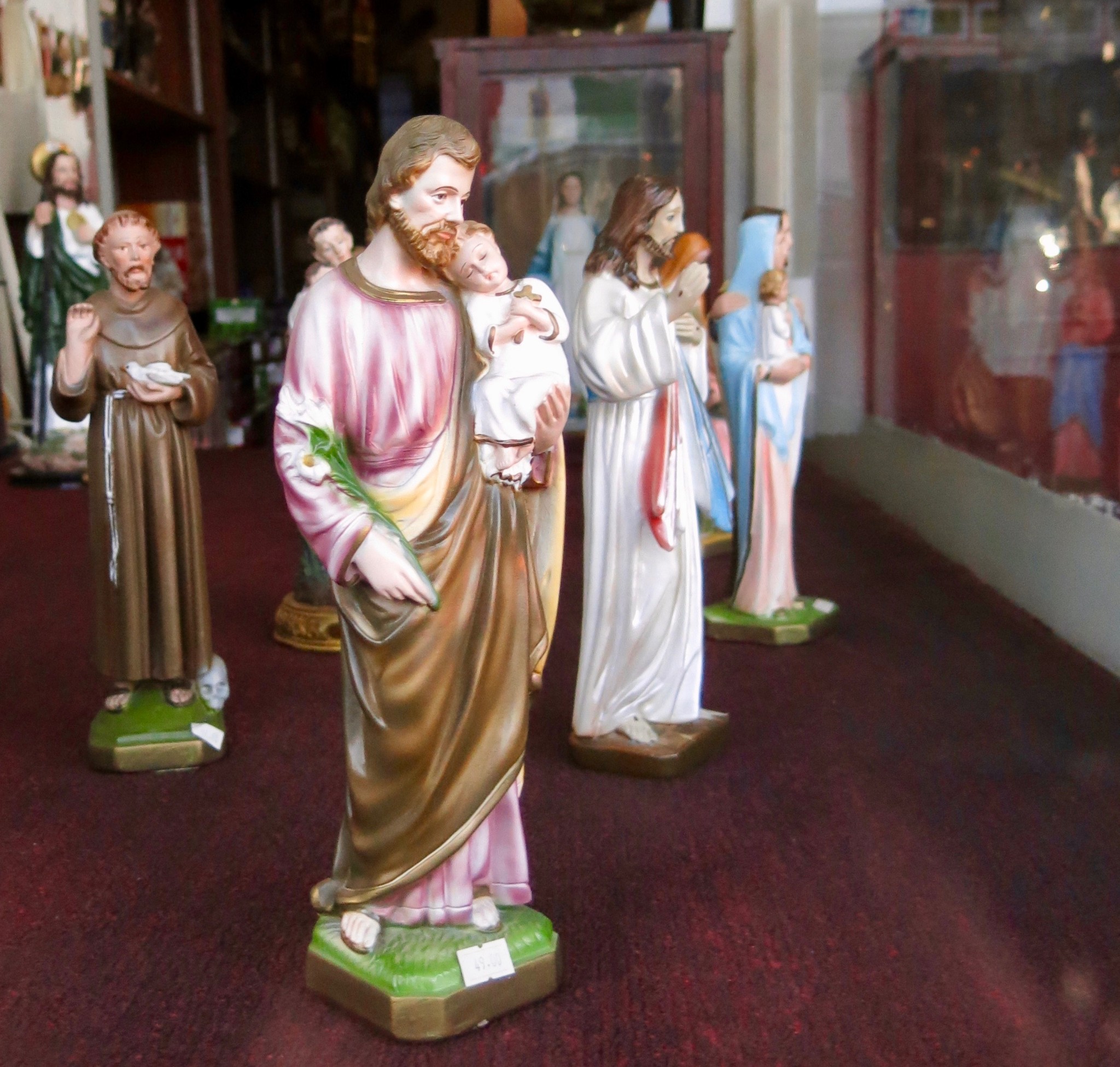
[436,27,739,321]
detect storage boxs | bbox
[121,201,290,452]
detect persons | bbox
[26,114,812,955]
